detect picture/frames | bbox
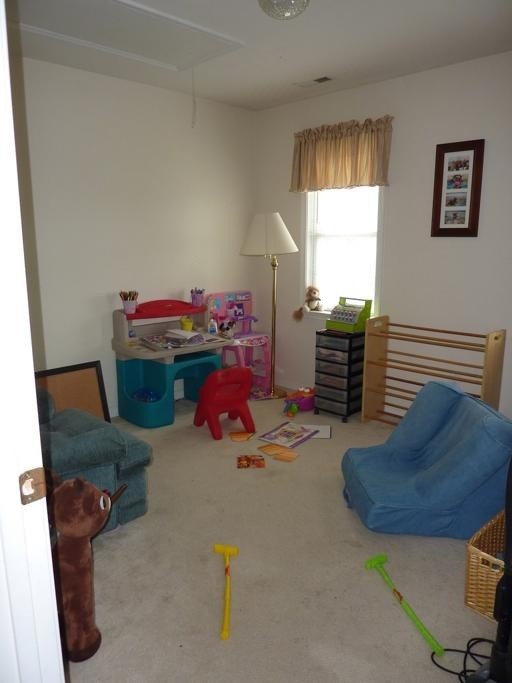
[429,138,485,238]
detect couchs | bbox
[36,390,154,536]
[340,379,512,541]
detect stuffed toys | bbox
[219,321,236,338]
[44,465,112,663]
[292,286,324,322]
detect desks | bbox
[110,299,234,429]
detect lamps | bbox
[238,210,300,400]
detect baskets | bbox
[464,510,506,623]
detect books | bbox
[257,421,320,448]
[302,424,330,439]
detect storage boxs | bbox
[458,511,509,624]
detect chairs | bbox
[192,366,257,440]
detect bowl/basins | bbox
[181,317,193,331]
